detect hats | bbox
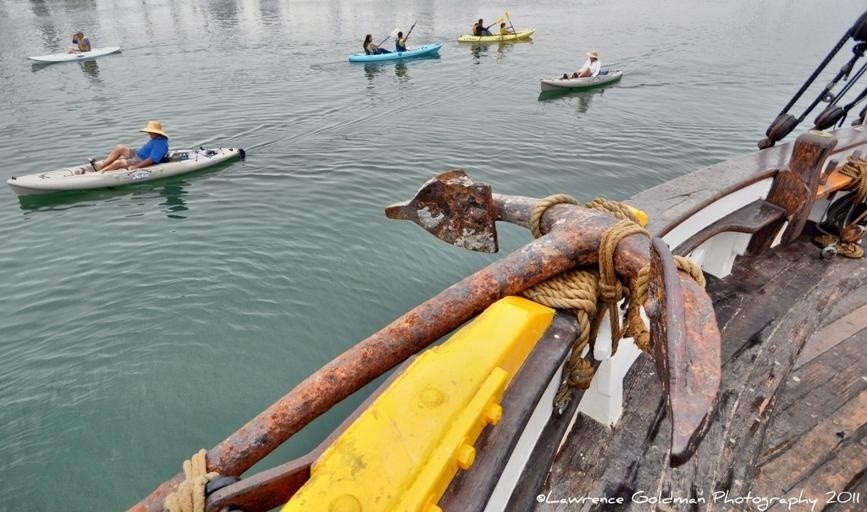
[139,120,170,140]
[585,50,598,60]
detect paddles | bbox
[504,10,517,34]
[375,28,399,47]
[486,18,505,29]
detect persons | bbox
[473,19,493,36]
[396,31,410,51]
[79,122,168,176]
[575,51,600,78]
[500,23,513,35]
[68,32,90,54]
[363,35,392,55]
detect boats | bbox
[539,70,623,95]
[457,28,536,44]
[5,145,245,199]
[25,45,120,66]
[346,43,444,65]
